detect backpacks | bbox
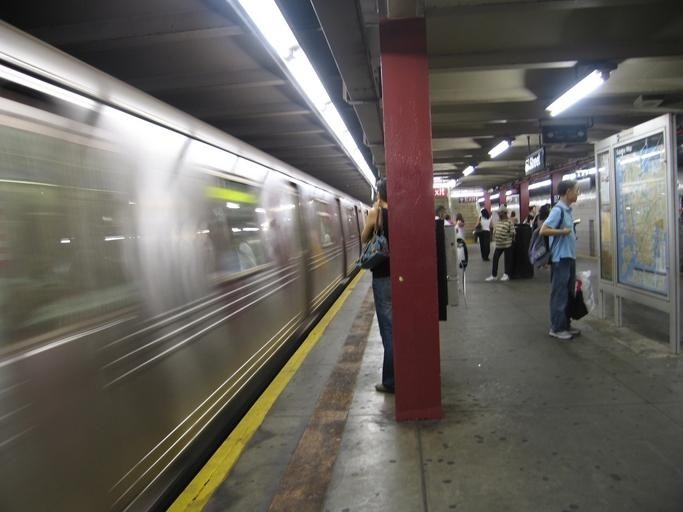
[528,205,564,269]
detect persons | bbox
[233,236,262,270]
[540,180,580,340]
[360,180,396,391]
[436,203,553,281]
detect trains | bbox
[0,12,380,511]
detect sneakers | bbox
[500,273,510,282]
[484,275,498,283]
[375,384,395,394]
[482,256,490,261]
[549,326,581,340]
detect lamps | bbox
[461,134,512,177]
[543,61,610,118]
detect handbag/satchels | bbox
[571,280,589,320]
[574,270,596,313]
[472,216,483,238]
[358,207,390,270]
